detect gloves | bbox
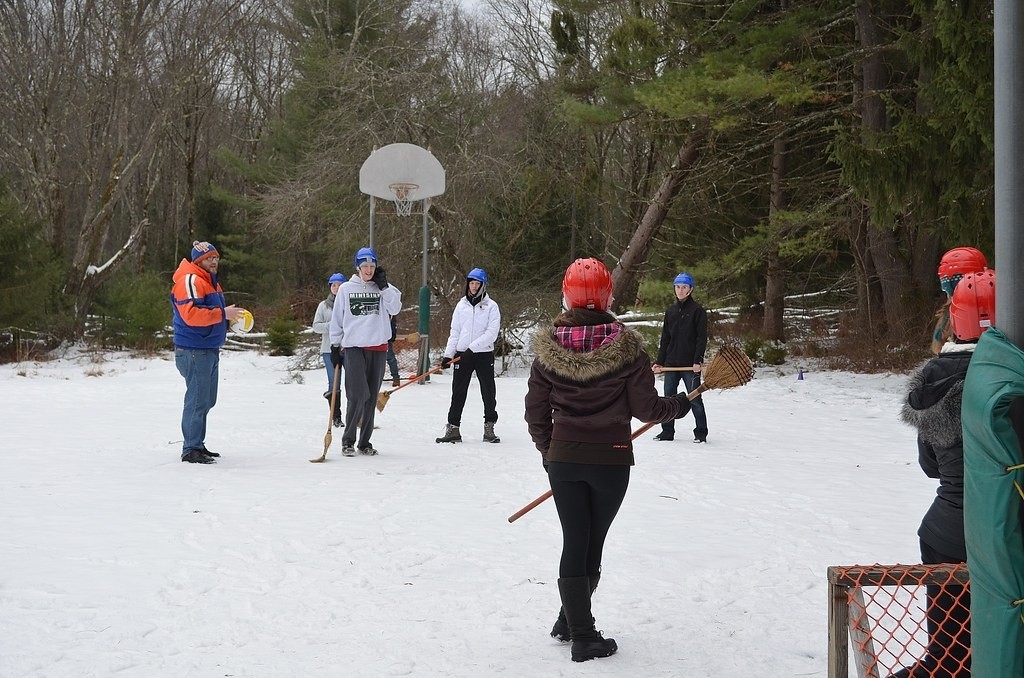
[460,348,473,366]
[671,392,691,419]
[330,345,343,369]
[441,357,452,369]
[373,266,389,291]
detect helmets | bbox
[354,248,378,269]
[672,272,694,291]
[938,246,988,280]
[326,273,347,288]
[561,258,614,312]
[949,269,995,341]
[467,268,487,285]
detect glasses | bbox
[205,255,221,261]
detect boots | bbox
[557,576,618,663]
[435,423,462,443]
[551,571,605,643]
[483,421,501,443]
[323,390,345,428]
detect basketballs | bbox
[229,309,254,334]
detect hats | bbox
[934,273,967,342]
[191,240,219,265]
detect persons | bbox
[312,273,346,428]
[525,257,691,661]
[328,247,402,457]
[889,267,998,678]
[652,272,708,443]
[436,268,500,444]
[386,314,400,387]
[170,240,244,464]
[931,246,988,357]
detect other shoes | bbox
[358,443,378,455]
[392,378,401,387]
[199,444,220,458]
[693,434,707,443]
[653,431,674,441]
[182,448,215,464]
[341,440,355,456]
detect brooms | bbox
[375,357,462,412]
[651,364,710,376]
[309,363,339,464]
[508,343,756,524]
